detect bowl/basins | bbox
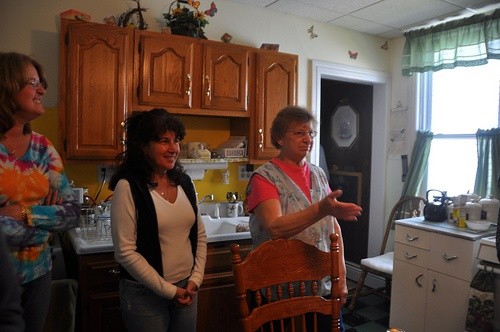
[465,220,492,230]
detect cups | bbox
[228,203,243,218]
[187,142,203,158]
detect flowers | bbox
[167,0,218,25]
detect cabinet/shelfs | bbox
[57,18,134,166]
[389,216,500,332]
[60,233,252,332]
[231,48,299,165]
[133,29,249,118]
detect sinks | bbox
[201,214,248,243]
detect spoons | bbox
[227,192,236,203]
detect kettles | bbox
[423,189,448,222]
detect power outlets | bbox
[238,166,250,179]
[97,164,114,182]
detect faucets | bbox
[196,193,214,206]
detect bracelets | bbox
[20,206,28,222]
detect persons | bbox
[246,106,363,332]
[108,108,207,332]
[0,51,84,332]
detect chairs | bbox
[348,197,428,313]
[229,233,340,332]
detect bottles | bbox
[448,190,500,222]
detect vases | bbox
[170,21,199,37]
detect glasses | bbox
[28,79,37,86]
[286,128,318,138]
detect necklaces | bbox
[153,185,171,202]
